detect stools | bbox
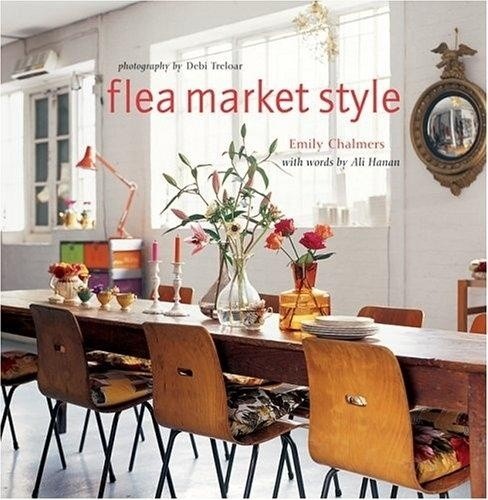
[1,344,69,471]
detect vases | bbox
[275,282,333,332]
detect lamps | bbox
[78,147,141,240]
[289,0,340,65]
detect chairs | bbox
[26,301,176,497]
[76,285,201,472]
[469,310,486,335]
[213,289,294,481]
[140,316,305,499]
[359,303,423,331]
[302,334,473,497]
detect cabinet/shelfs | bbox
[57,241,146,297]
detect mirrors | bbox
[408,25,484,199]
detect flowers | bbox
[48,262,137,314]
[153,122,295,328]
[263,219,339,290]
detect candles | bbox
[172,234,183,262]
[151,240,160,260]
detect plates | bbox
[298,314,381,341]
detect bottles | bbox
[63,201,77,228]
[80,200,94,229]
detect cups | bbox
[75,287,96,308]
[96,291,117,311]
[239,306,274,332]
[115,291,138,312]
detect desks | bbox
[1,285,487,496]
